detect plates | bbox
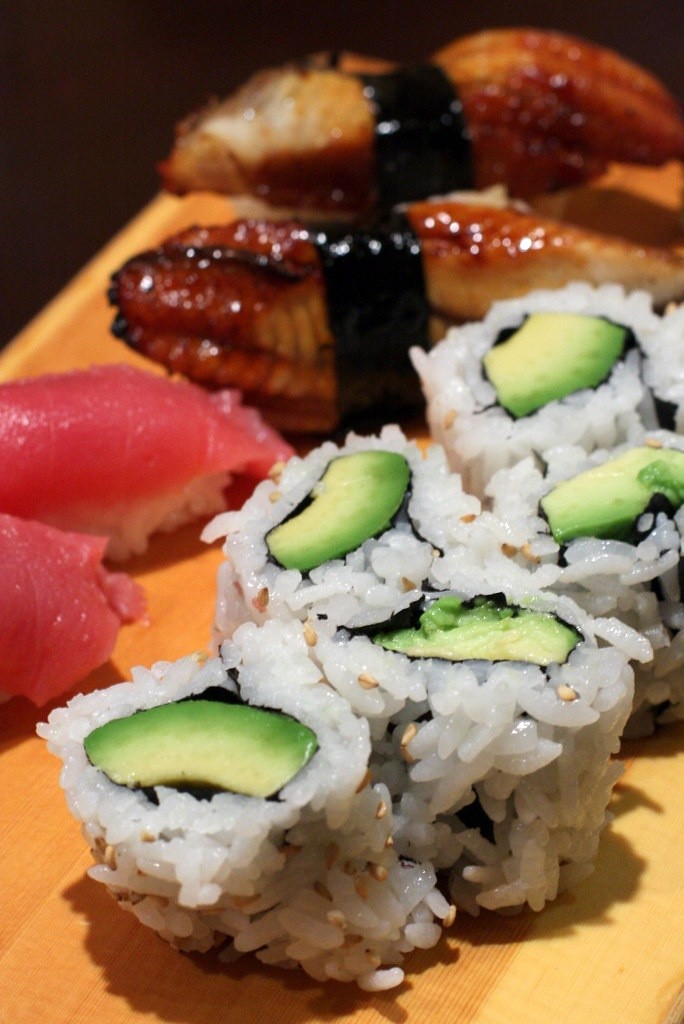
[0,194,682,1022]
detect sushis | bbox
[1,29,684,989]
